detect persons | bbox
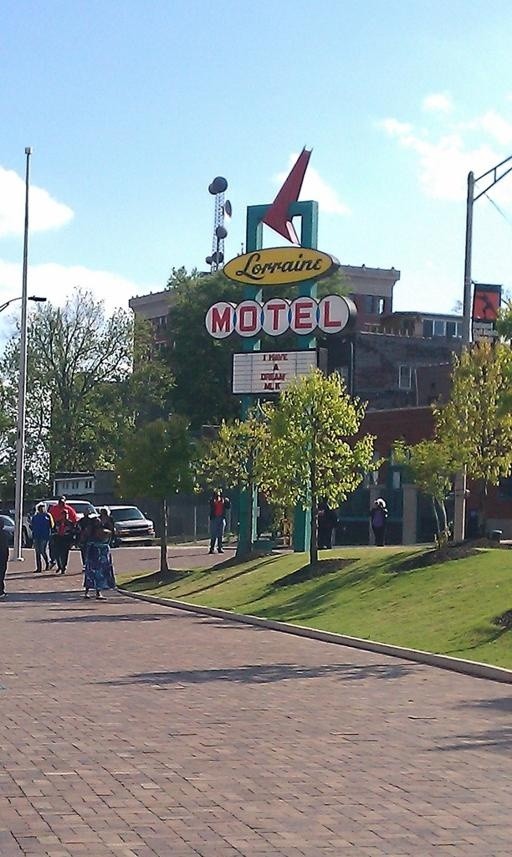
[30,496,116,600]
[369,496,389,545]
[318,499,338,549]
[0,517,10,599]
[208,488,232,554]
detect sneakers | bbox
[83,593,90,600]
[96,593,107,602]
[218,547,225,553]
[209,546,215,554]
[30,560,69,576]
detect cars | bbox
[2,495,157,550]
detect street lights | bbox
[7,142,34,563]
[0,294,48,311]
